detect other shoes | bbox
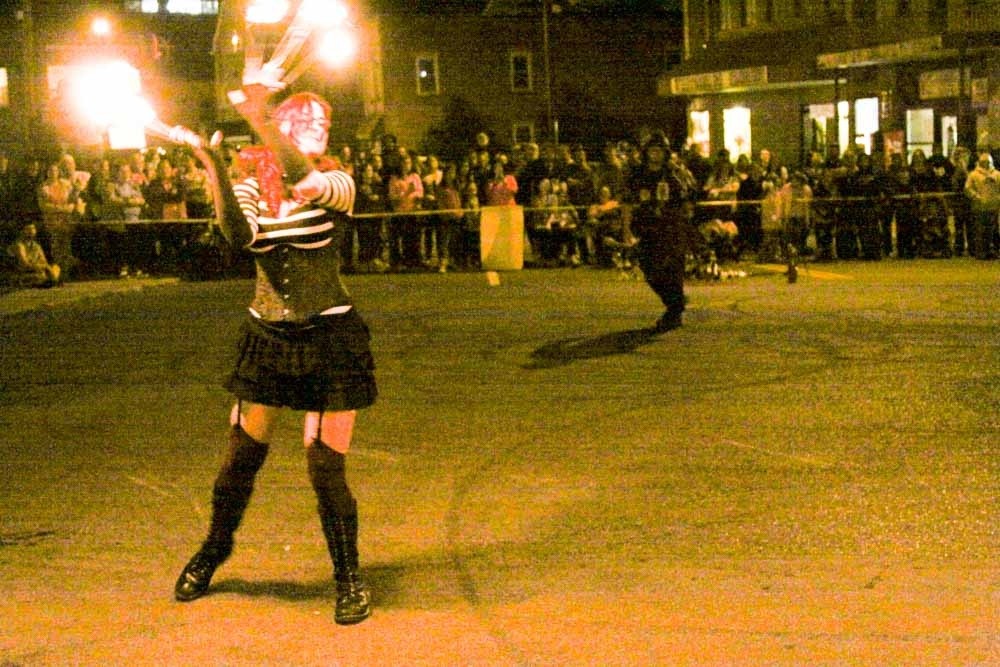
[136,269,147,279]
[119,266,129,277]
[656,314,681,332]
[341,250,637,275]
[789,271,798,284]
[792,246,1000,264]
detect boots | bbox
[317,498,371,623]
[174,484,251,600]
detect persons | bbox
[169,52,378,624]
[630,129,689,329]
[0,141,264,287]
[671,131,1000,280]
[325,130,638,276]
